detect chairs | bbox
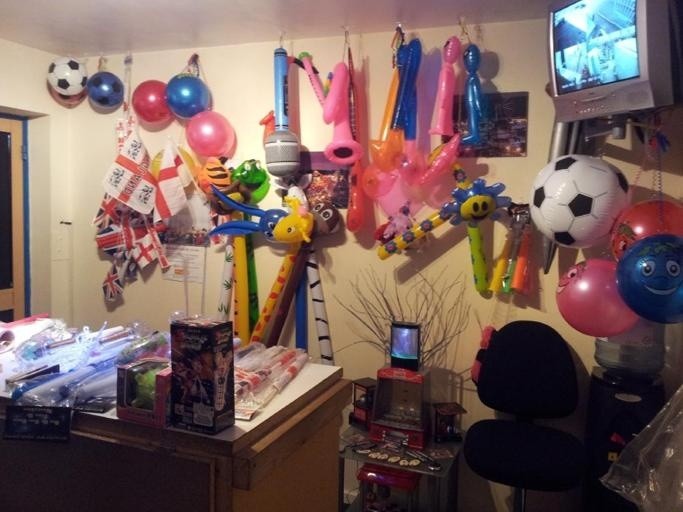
[462,319,589,510]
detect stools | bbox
[356,462,423,511]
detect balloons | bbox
[348,132,536,295]
[378,36,490,147]
[259,46,366,234]
[199,160,341,350]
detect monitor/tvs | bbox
[546,0,683,123]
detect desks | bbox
[337,424,460,511]
[0,362,353,511]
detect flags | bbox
[89,126,193,307]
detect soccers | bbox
[86,72,124,105]
[46,57,87,97]
[528,153,629,251]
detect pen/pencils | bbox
[406,449,433,462]
